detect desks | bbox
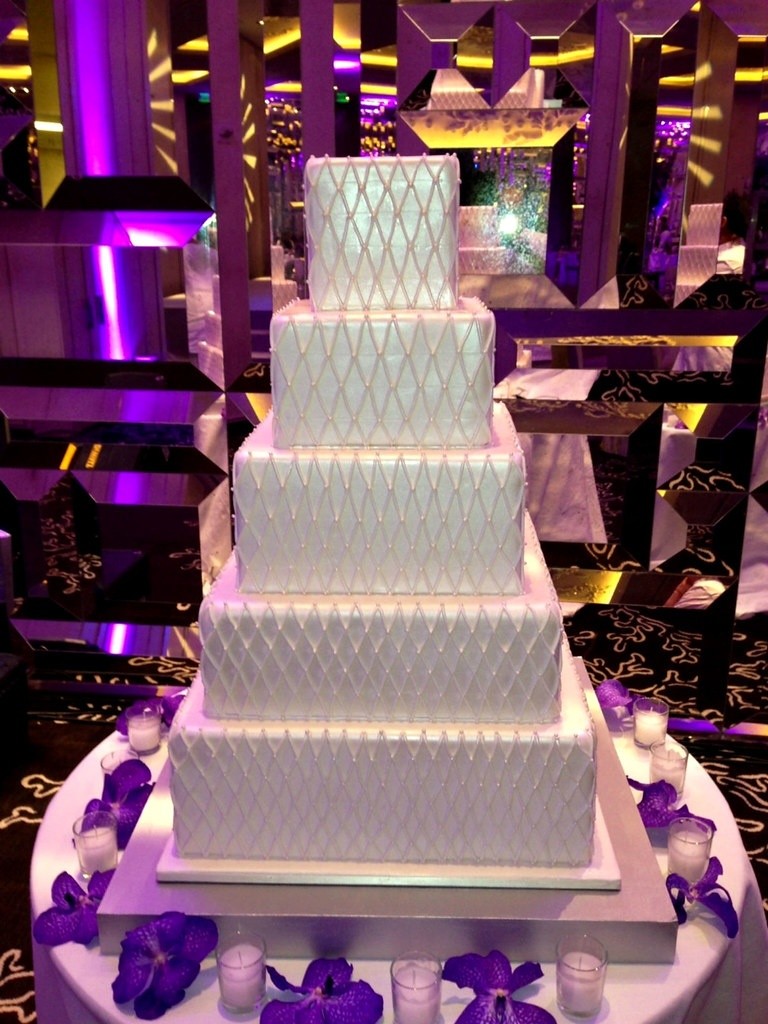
[33,689,768,1023]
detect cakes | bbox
[165,152,597,866]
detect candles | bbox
[221,943,264,1008]
[637,706,666,746]
[653,743,685,794]
[668,828,710,884]
[129,708,158,752]
[393,964,440,1024]
[80,823,118,874]
[560,951,602,1012]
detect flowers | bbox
[110,911,220,1020]
[32,867,120,943]
[626,774,719,835]
[666,856,740,939]
[111,688,190,737]
[440,950,560,1024]
[71,758,156,852]
[259,956,385,1024]
[596,679,666,715]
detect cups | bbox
[648,739,688,794]
[633,698,669,749]
[556,932,607,1020]
[216,930,266,1014]
[390,950,442,1024]
[668,817,713,882]
[125,705,161,754]
[73,811,117,878]
[101,751,138,774]
